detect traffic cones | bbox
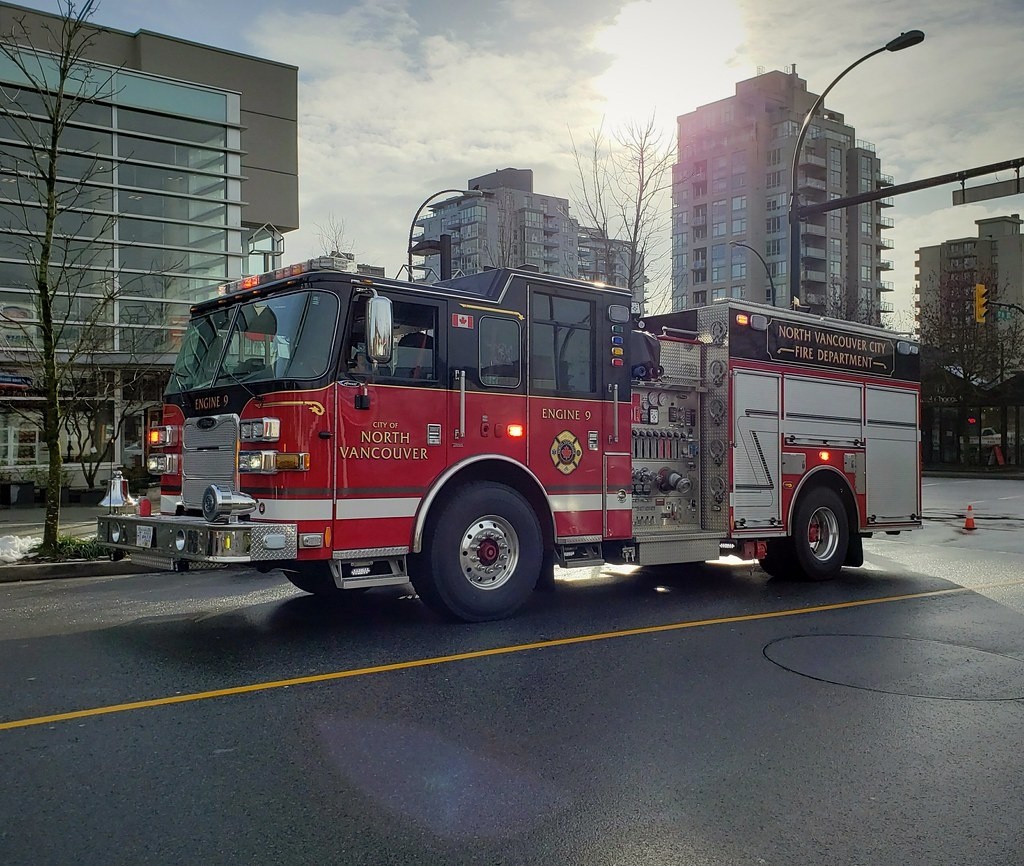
[962,504,979,529]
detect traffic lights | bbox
[790,295,799,311]
[976,284,990,323]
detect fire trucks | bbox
[93,249,925,627]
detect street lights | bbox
[408,189,484,282]
[728,241,775,306]
[788,29,925,306]
